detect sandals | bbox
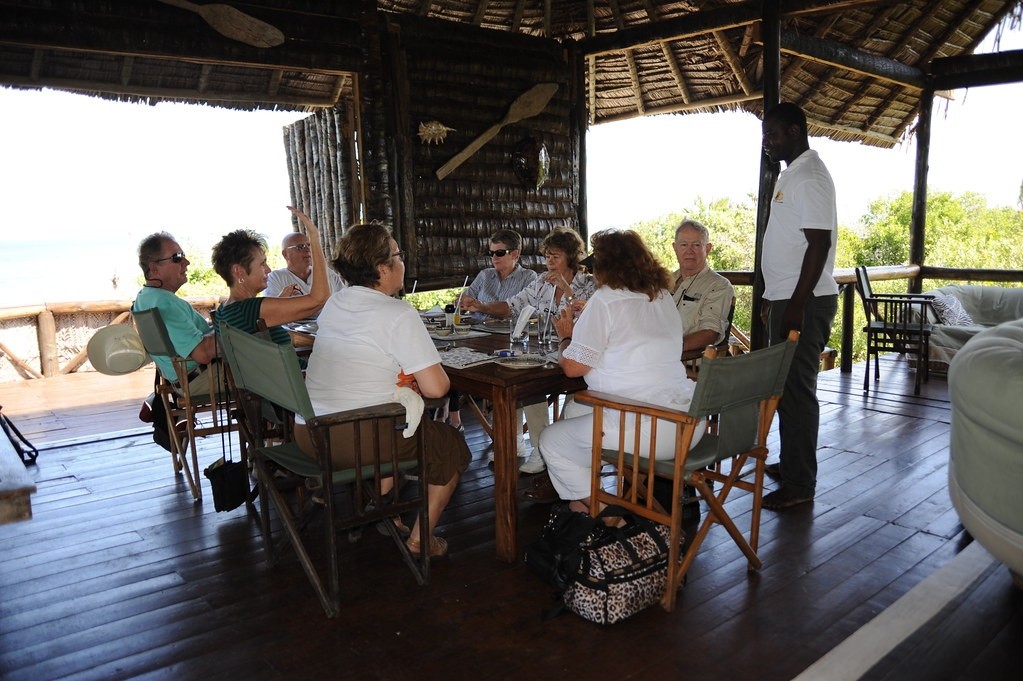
[456,423,465,435]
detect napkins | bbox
[513,305,535,338]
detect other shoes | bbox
[251,463,288,481]
[376,515,410,538]
[403,536,448,562]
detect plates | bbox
[494,356,551,369]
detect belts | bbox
[165,358,222,390]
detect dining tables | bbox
[295,330,703,563]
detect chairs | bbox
[855,266,936,397]
[130,297,803,619]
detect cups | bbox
[510,343,530,355]
[455,323,471,335]
[446,313,460,334]
[406,294,419,310]
[435,328,452,336]
[538,312,552,344]
[510,320,530,342]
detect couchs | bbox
[899,284,1023,373]
[948,320,1023,577]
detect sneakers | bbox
[489,438,526,461]
[519,448,547,473]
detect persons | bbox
[757,102,838,509]
[534,230,708,528]
[131,205,344,423]
[455,221,736,473]
[294,224,471,559]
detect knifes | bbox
[462,357,500,366]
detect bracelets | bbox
[559,336,571,346]
[568,295,576,300]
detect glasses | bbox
[391,250,407,261]
[155,253,185,262]
[487,248,514,257]
[286,243,311,252]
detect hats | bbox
[87,323,153,376]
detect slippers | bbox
[536,508,590,542]
[578,519,641,550]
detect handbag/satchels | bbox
[203,457,251,513]
[151,395,197,454]
[562,504,688,626]
[623,471,700,527]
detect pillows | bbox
[929,294,975,327]
